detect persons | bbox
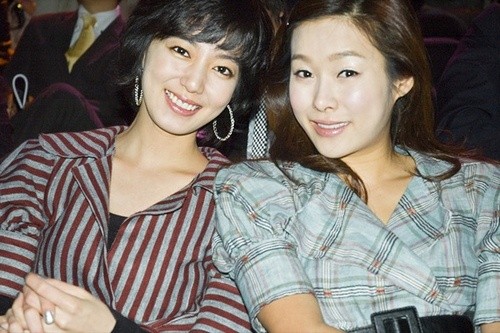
[0,0,274,333]
[0,0,500,176]
[212,1,500,333]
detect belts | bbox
[344,307,476,333]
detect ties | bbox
[64,14,96,74]
